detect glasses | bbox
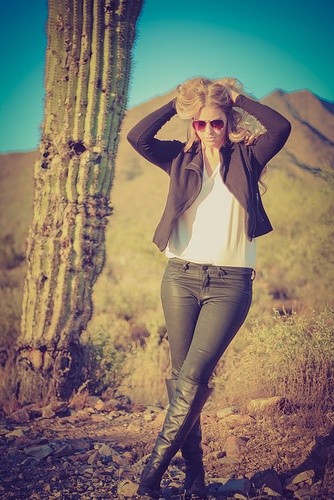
[192,118,226,131]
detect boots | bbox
[137,379,214,500]
[166,379,206,498]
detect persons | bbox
[127,78,291,500]
